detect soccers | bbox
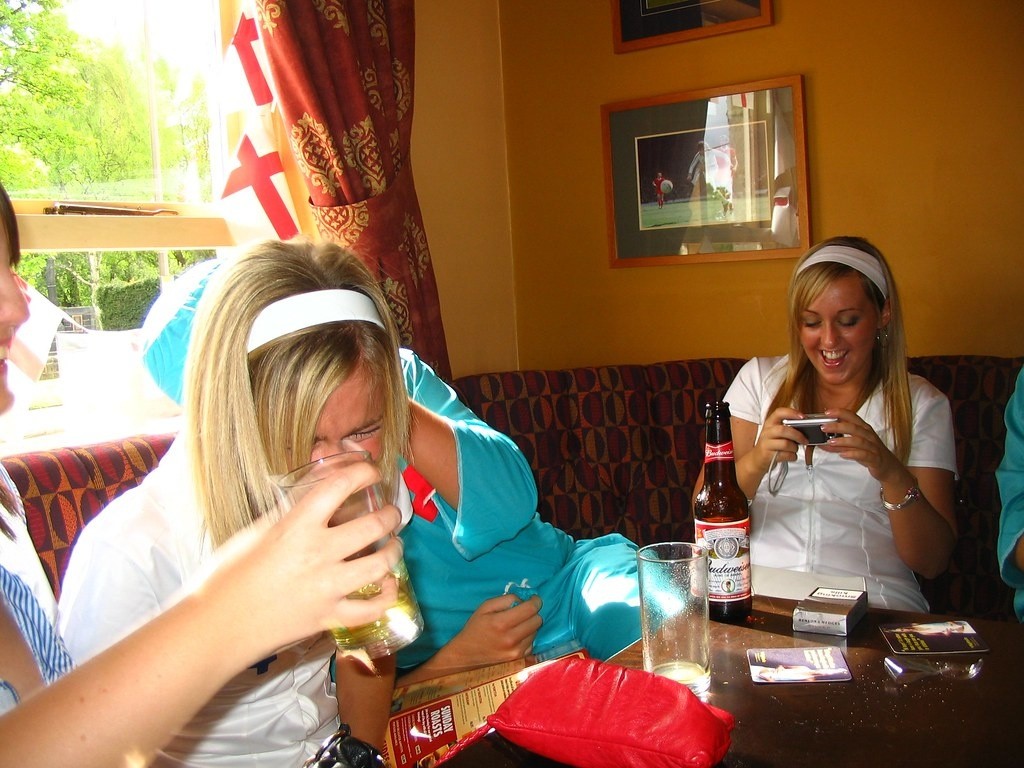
[661,179,673,193]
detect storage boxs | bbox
[376,639,589,768]
[793,587,868,635]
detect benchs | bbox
[0,352,1023,659]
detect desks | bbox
[606,584,1024,768]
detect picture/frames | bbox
[609,0,776,55]
[599,72,811,271]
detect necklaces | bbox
[817,387,867,412]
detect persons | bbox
[653,172,666,208]
[687,141,729,215]
[137,256,686,688]
[0,184,58,617]
[55,240,417,768]
[692,235,960,614]
[995,363,1024,626]
[0,462,404,767]
[715,135,738,215]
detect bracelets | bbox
[880,474,921,510]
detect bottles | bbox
[694,401,753,624]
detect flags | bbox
[223,0,281,119]
[8,270,62,383]
[219,119,300,247]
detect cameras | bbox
[782,413,844,445]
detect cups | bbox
[277,450,425,661]
[637,542,713,695]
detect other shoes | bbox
[660,205,663,209]
[729,202,732,213]
[723,201,729,212]
